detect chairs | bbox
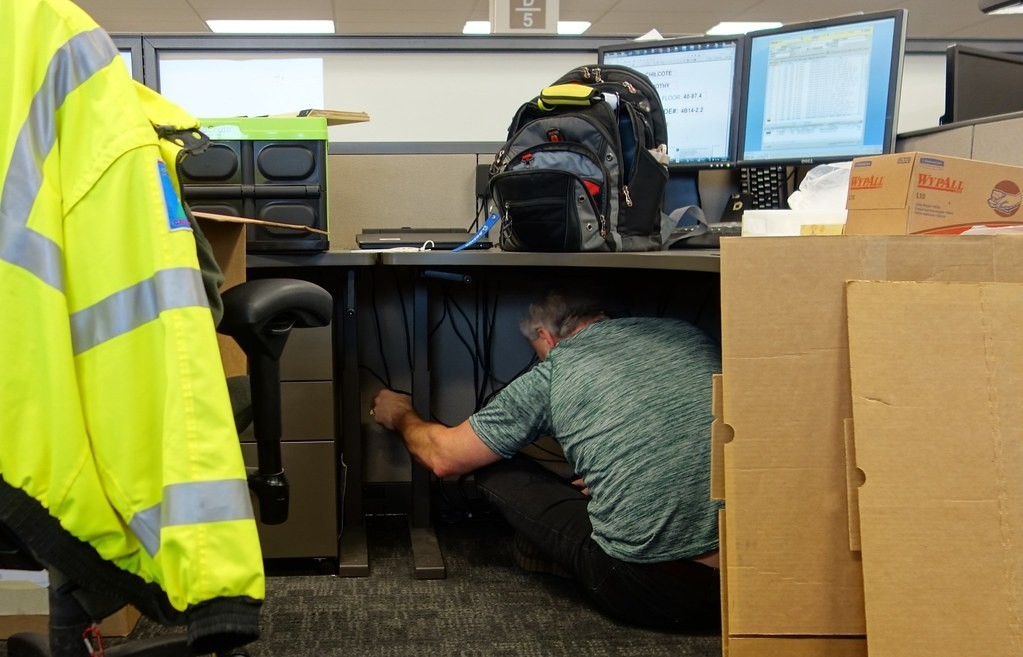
[0,0,339,657]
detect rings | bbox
[370,409,375,417]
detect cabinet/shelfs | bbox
[234,321,338,568]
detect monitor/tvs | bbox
[598,33,745,169]
[734,9,909,169]
[945,44,1023,124]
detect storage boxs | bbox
[1,575,143,642]
[845,151,1023,235]
[192,208,330,376]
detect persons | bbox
[369,274,722,633]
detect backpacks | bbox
[489,64,668,251]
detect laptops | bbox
[356,231,494,250]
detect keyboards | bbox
[669,221,742,249]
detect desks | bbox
[233,249,719,581]
